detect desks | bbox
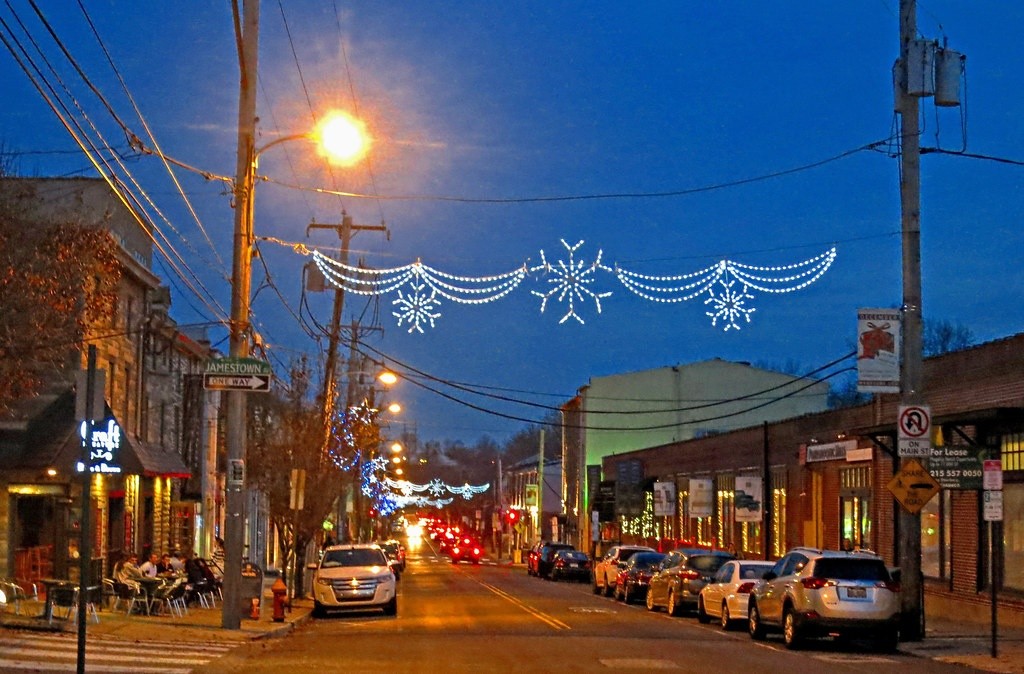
[31,578,67,626]
[135,578,162,615]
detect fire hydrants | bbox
[270,577,294,625]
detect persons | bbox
[112,551,215,615]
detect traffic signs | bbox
[202,357,272,392]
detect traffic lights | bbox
[506,509,518,524]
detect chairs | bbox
[0,565,224,626]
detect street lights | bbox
[301,367,411,589]
[223,105,373,634]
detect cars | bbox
[419,518,483,563]
[312,545,397,618]
[593,544,905,649]
[373,541,406,579]
[527,539,592,581]
